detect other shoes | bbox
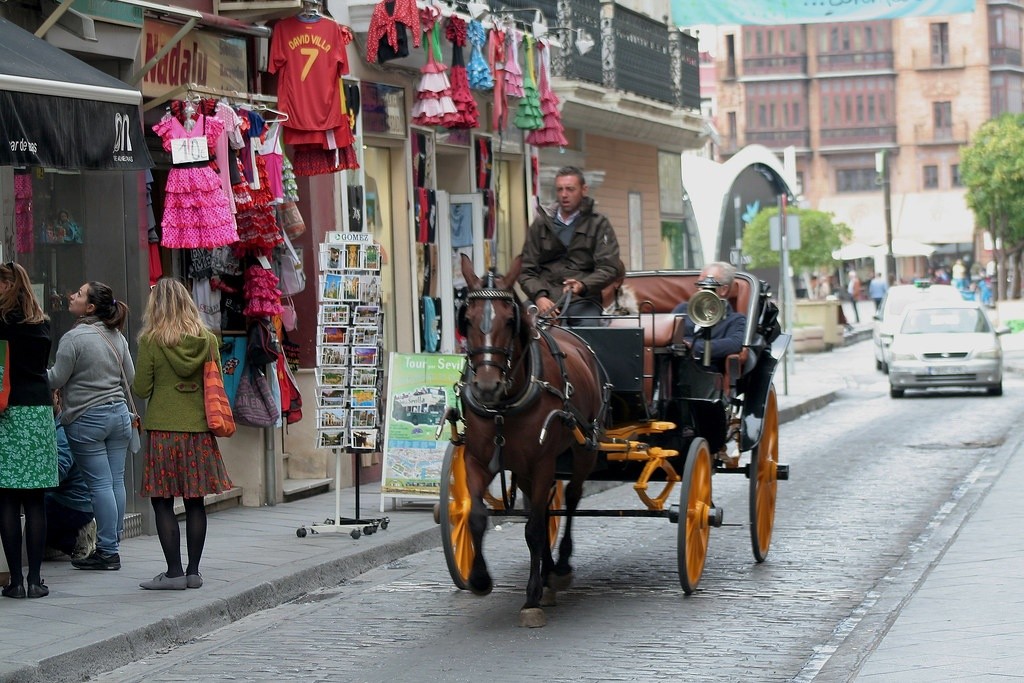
[715,451,733,467]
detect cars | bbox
[878,302,1011,400]
[872,279,967,369]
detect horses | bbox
[458,252,605,625]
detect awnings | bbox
[0,13,156,176]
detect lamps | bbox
[492,7,548,38]
[548,27,595,57]
[450,0,490,20]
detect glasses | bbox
[6,260,16,277]
[150,285,156,291]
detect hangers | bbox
[299,0,323,15]
[179,83,289,123]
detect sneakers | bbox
[2,584,26,598]
[187,572,204,588]
[71,550,122,571]
[140,572,187,590]
[27,579,49,598]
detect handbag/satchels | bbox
[129,412,143,453]
[279,228,306,298]
[233,368,280,428]
[282,296,296,332]
[204,344,237,438]
[0,339,10,411]
[280,201,305,241]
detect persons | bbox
[0,260,56,599]
[132,278,222,591]
[847,270,861,322]
[807,273,840,299]
[668,262,747,455]
[926,257,997,307]
[869,272,888,310]
[47,388,96,560]
[46,281,138,570]
[516,168,625,329]
[600,259,639,316]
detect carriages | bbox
[433,250,790,630]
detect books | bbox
[316,242,382,448]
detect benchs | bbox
[606,314,686,401]
[615,277,751,402]
